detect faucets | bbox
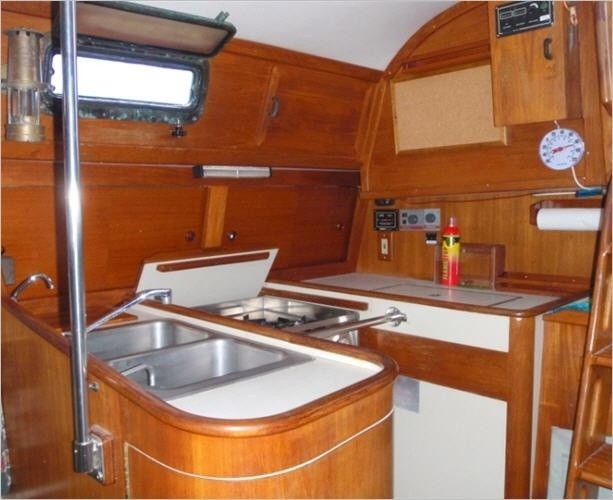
[12,273,55,299]
[87,288,174,337]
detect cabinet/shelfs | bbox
[564,173,611,500]
[487,0,581,128]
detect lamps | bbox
[192,161,273,183]
[2,26,52,144]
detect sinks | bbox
[109,339,315,400]
[82,318,213,361]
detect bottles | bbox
[439,216,461,285]
